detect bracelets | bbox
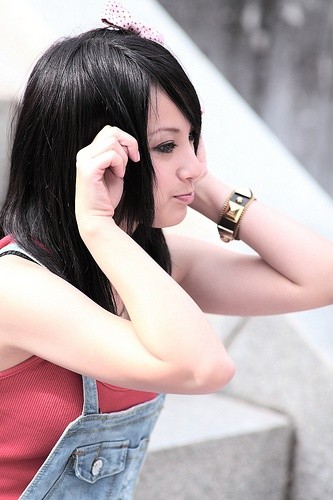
[218,185,257,243]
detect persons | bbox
[0,29,333,500]
